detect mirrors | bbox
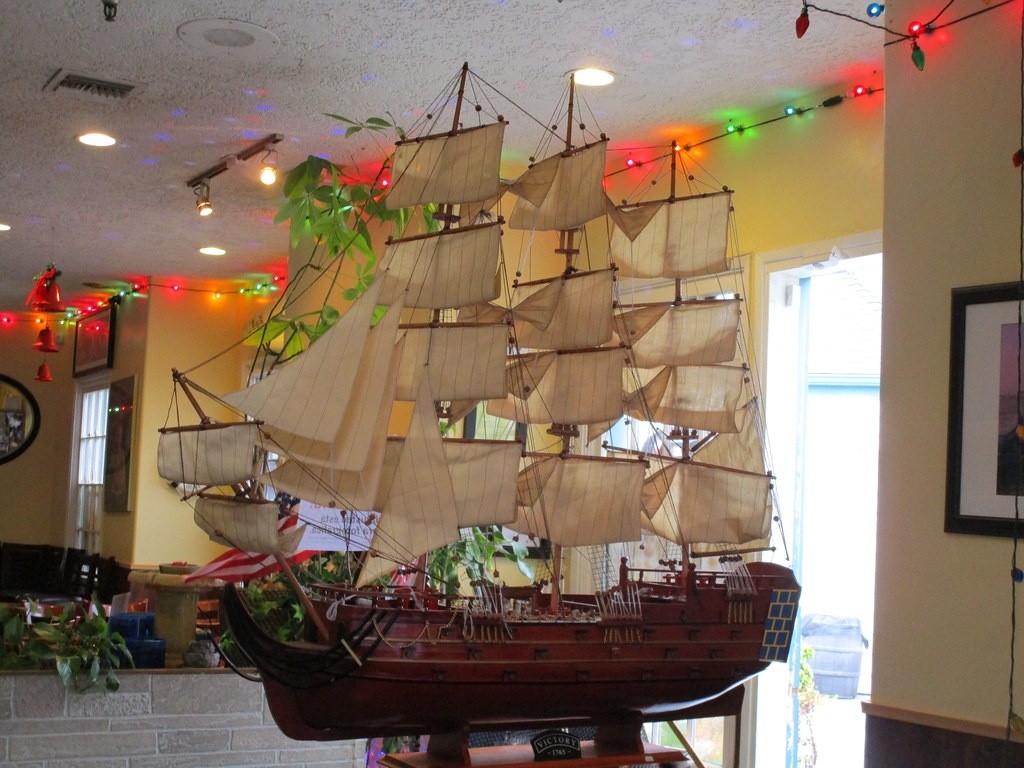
[0,373,41,465]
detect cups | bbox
[126,638,165,668]
[107,612,155,647]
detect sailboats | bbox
[158,61,802,742]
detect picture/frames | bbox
[72,306,117,378]
[945,278,1024,540]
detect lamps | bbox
[260,160,278,186]
[196,197,213,217]
